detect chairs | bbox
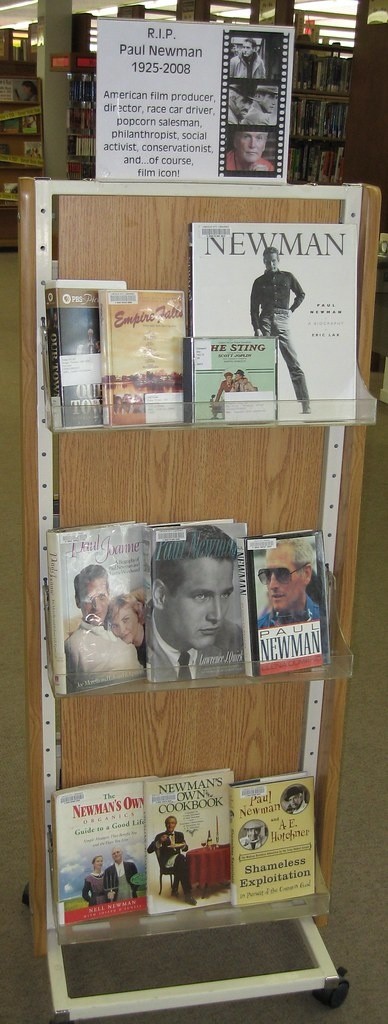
[155,847,174,895]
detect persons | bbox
[216,247,310,416]
[66,526,324,691]
[22,115,36,129]
[82,816,197,907]
[229,39,275,171]
[18,81,38,101]
[284,787,307,814]
[53,312,100,414]
[239,821,267,849]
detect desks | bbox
[186,844,231,899]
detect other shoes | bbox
[185,897,197,905]
[171,891,180,897]
[303,402,311,414]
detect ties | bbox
[178,651,193,682]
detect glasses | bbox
[257,563,309,586]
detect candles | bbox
[216,817,218,832]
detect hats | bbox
[257,85,280,96]
[243,821,263,830]
[234,370,245,375]
[230,83,262,102]
[284,787,304,802]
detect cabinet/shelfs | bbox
[18,176,382,1024]
[0,0,388,373]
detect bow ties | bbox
[169,834,174,836]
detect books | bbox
[51,777,156,925]
[230,771,316,907]
[289,52,352,185]
[45,281,127,430]
[143,519,247,681]
[144,768,237,914]
[69,75,96,180]
[237,529,330,676]
[187,222,355,422]
[98,290,184,427]
[11,40,29,62]
[46,521,149,694]
[183,336,277,424]
